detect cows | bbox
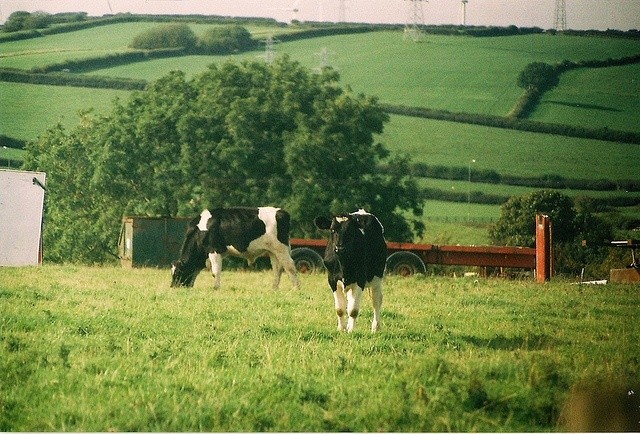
[314,209,388,333]
[171,207,301,291]
